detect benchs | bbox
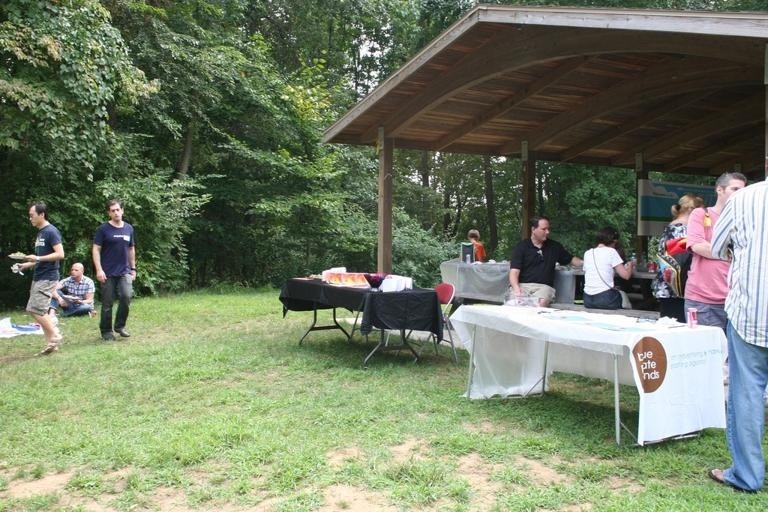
[550,303,661,319]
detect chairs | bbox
[395,283,459,365]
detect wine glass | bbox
[362,271,388,292]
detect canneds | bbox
[465,254,471,265]
[648,261,657,273]
[688,307,698,327]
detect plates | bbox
[7,254,27,259]
[62,294,81,302]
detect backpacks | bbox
[656,241,691,298]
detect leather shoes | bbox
[114,327,130,337]
[102,331,115,341]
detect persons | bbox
[13,203,65,358]
[649,195,706,323]
[682,172,749,334]
[467,229,486,262]
[503,215,584,308]
[710,152,766,492]
[53,262,95,317]
[582,227,635,309]
[91,199,138,342]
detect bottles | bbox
[465,254,471,264]
[630,252,638,270]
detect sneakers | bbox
[710,468,733,483]
[33,336,63,356]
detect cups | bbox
[488,260,495,264]
[647,261,656,274]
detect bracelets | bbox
[36,256,40,264]
[131,268,136,270]
